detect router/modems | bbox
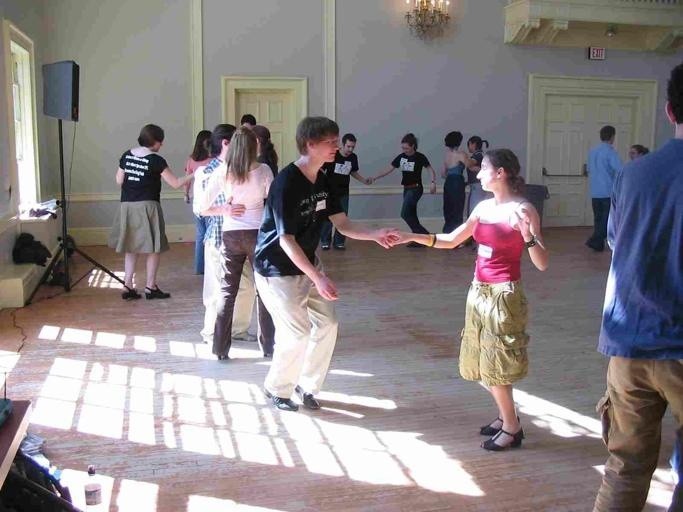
[0,372,11,427]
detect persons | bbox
[184,115,278,362]
[386,149,549,451]
[107,124,194,299]
[253,117,400,411]
[319,131,489,250]
[585,126,624,252]
[629,145,649,160]
[591,58,683,512]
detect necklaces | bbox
[525,235,537,249]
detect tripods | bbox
[24,118,136,305]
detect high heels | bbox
[145,284,172,301]
[121,285,142,302]
[478,423,525,451]
[479,415,521,439]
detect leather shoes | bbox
[262,387,300,413]
[294,384,322,410]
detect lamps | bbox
[404,0,451,32]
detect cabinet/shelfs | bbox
[0,350,33,494]
[0,205,64,308]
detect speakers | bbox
[42,61,79,121]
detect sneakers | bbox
[198,324,276,362]
[322,238,346,253]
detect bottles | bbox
[83,464,103,512]
